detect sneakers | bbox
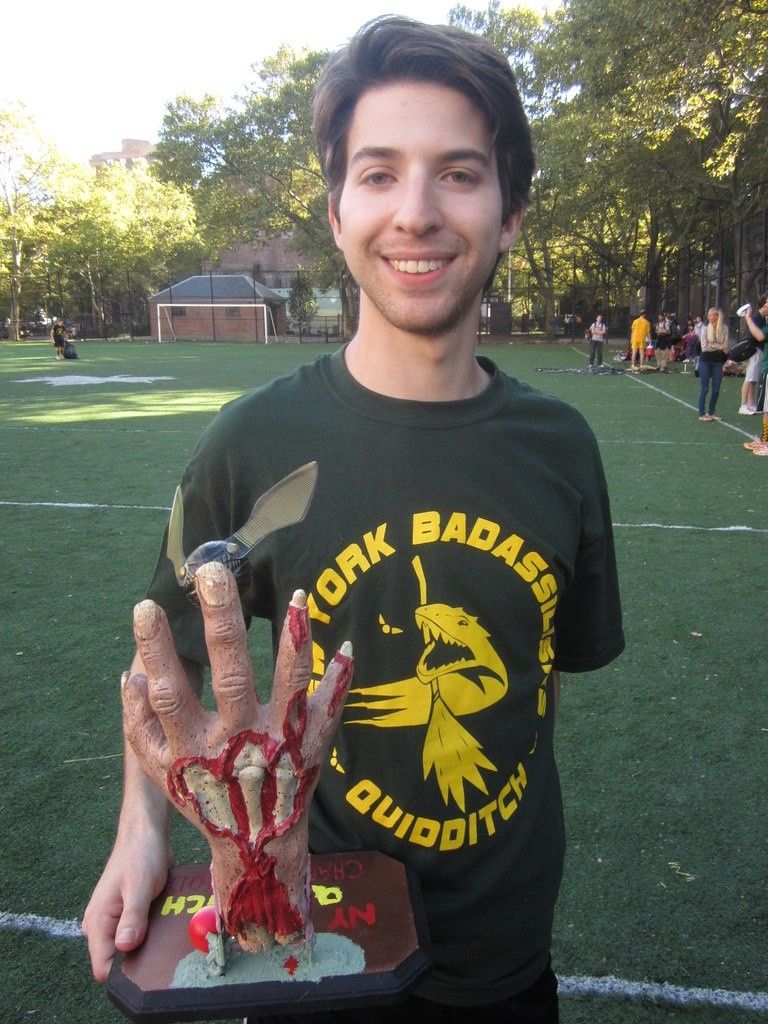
[748,406,763,414]
[753,446,768,456]
[744,438,768,450]
[738,407,755,416]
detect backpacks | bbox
[668,320,678,335]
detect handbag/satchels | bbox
[731,339,756,362]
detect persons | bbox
[738,295,768,456]
[698,307,728,422]
[555,313,585,344]
[588,315,607,367]
[83,10,626,1023]
[120,562,354,953]
[630,309,705,374]
[51,319,77,360]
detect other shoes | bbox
[589,365,593,368]
[708,415,722,421]
[598,365,605,368]
[683,359,690,363]
[56,354,64,360]
[698,415,713,421]
